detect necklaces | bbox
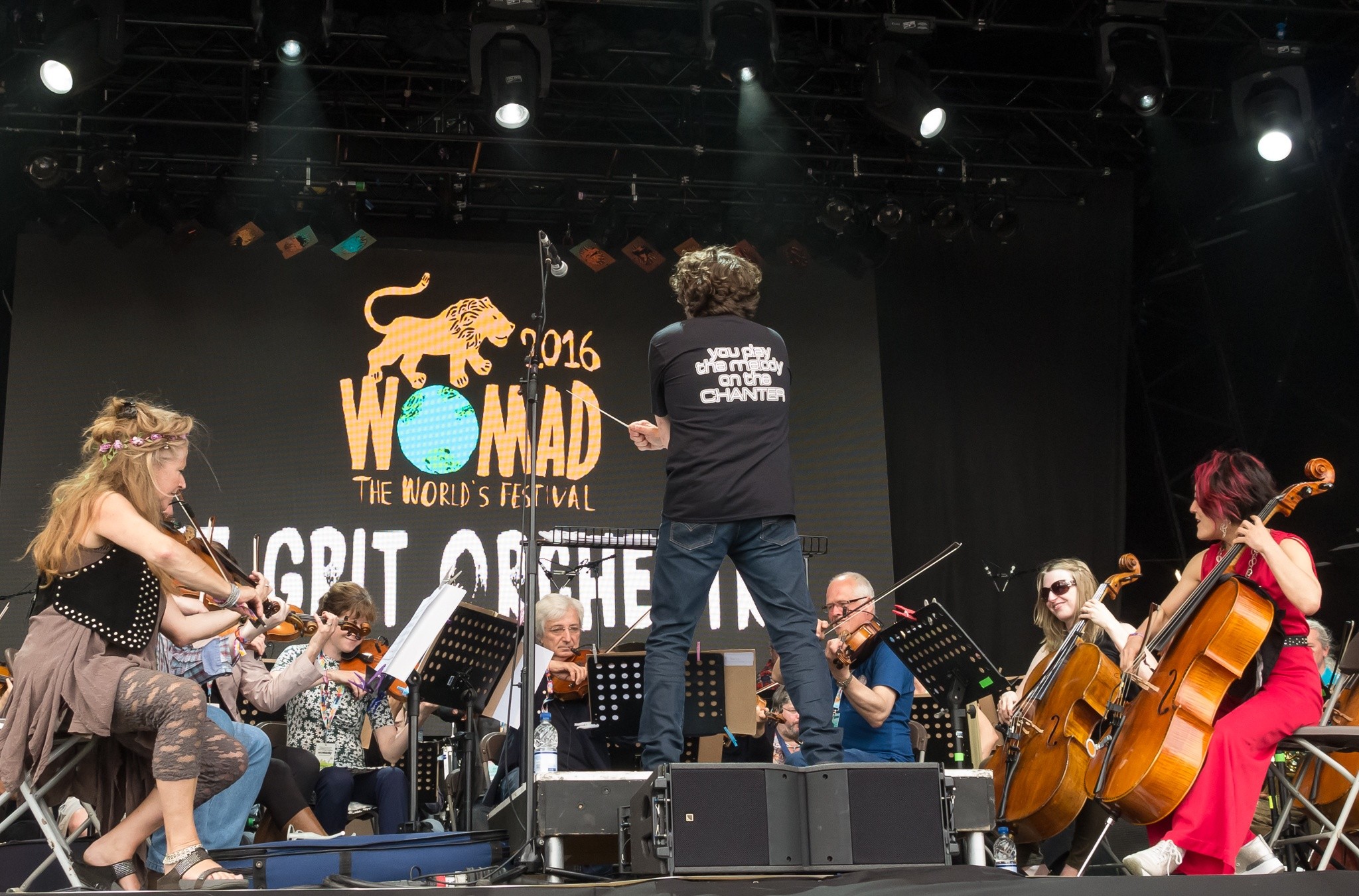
[1216,541,1259,580]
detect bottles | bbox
[534,712,558,773]
[544,836,565,884]
[993,827,1018,874]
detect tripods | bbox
[474,267,623,889]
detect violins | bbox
[191,536,283,618]
[756,693,787,724]
[215,603,372,642]
[832,618,883,670]
[0,661,14,698]
[154,511,241,588]
[338,637,422,703]
[550,648,592,702]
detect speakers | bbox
[630,765,950,873]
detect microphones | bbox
[538,231,570,279]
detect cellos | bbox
[977,553,1143,846]
[1076,457,1337,880]
[1290,671,1359,872]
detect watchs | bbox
[837,673,852,690]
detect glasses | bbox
[821,596,868,613]
[548,625,583,634]
[782,707,796,714]
[1040,579,1075,601]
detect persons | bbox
[271,583,438,834]
[996,558,1159,877]
[627,243,849,767]
[1248,617,1350,843]
[753,635,803,764]
[116,536,288,890]
[814,571,915,763]
[1119,449,1323,880]
[476,592,614,826]
[0,400,340,896]
[187,539,340,843]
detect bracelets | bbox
[1128,633,1144,640]
[214,582,240,609]
[234,630,252,657]
[322,669,328,683]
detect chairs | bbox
[0,730,101,896]
[1270,633,1359,872]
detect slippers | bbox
[71,852,145,892]
[157,846,249,890]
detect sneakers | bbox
[1123,840,1186,877]
[1234,834,1285,875]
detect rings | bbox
[998,707,1001,710]
[265,584,269,587]
[326,622,332,626]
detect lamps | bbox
[919,186,966,243]
[20,148,65,189]
[969,183,1020,245]
[560,220,810,273]
[1089,0,1172,117]
[39,0,125,95]
[251,0,336,69]
[861,12,950,144]
[1229,38,1315,165]
[469,0,553,133]
[859,190,911,240]
[85,148,131,195]
[696,0,780,88]
[813,193,855,237]
[88,189,376,261]
